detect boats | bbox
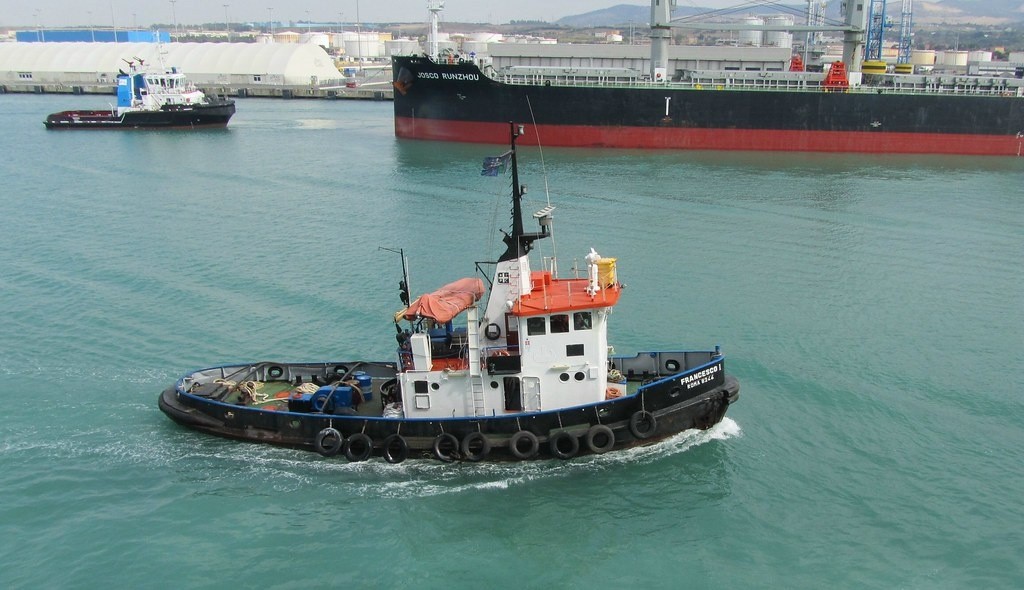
[157,114,744,464]
[382,0,1024,157]
[42,30,237,131]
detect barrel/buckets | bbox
[357,375,373,399]
[354,371,364,379]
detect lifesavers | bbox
[508,430,541,460]
[382,435,408,463]
[627,410,658,439]
[341,433,374,462]
[461,431,491,462]
[551,430,580,459]
[315,427,344,458]
[586,424,615,454]
[432,432,459,462]
[493,350,510,356]
